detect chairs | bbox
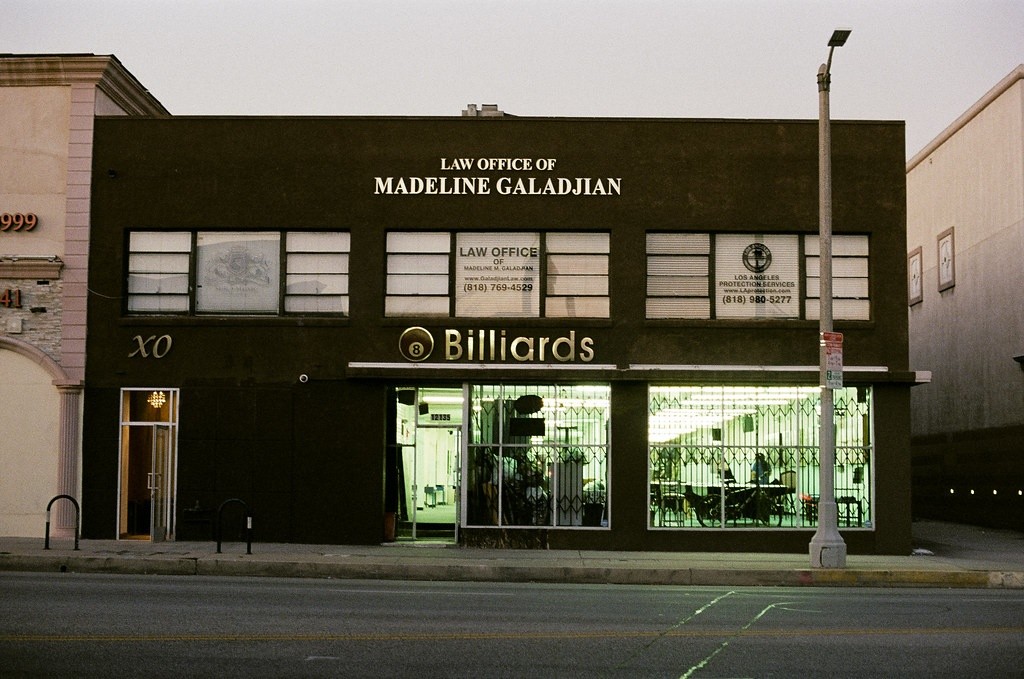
[658,477,685,527]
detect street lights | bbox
[808,29,852,567]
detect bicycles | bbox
[695,469,782,529]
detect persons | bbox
[750,453,772,484]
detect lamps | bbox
[147,391,166,409]
